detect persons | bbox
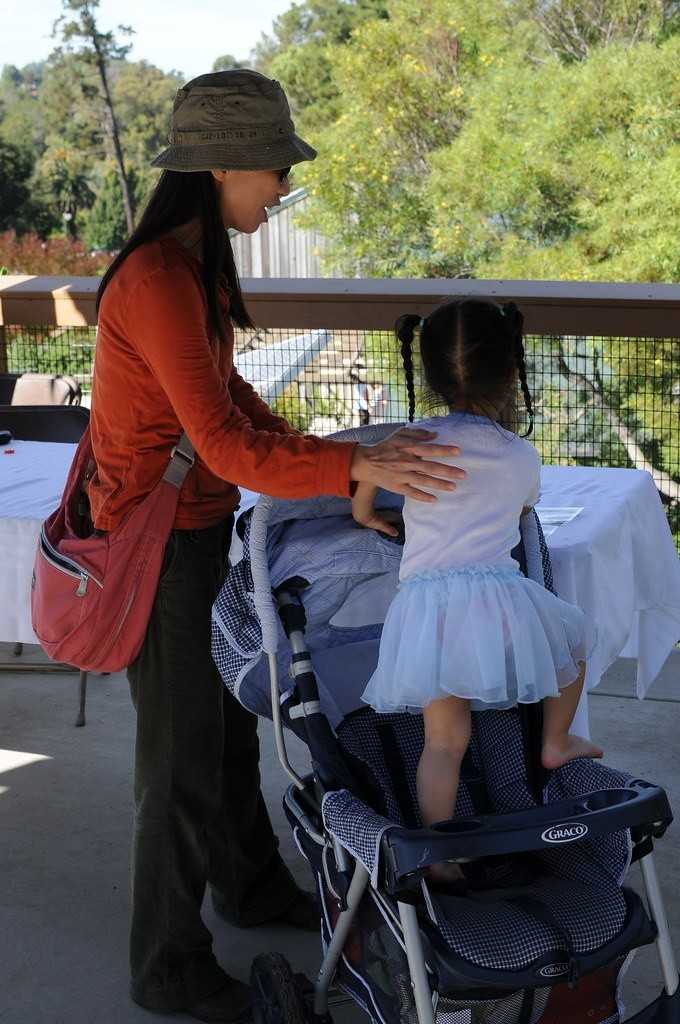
[88,69,467,1024]
[351,298,604,880]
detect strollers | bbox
[213,424,679,1024]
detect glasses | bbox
[272,166,291,182]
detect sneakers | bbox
[280,890,323,931]
[130,973,255,1022]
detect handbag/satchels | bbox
[31,423,191,673]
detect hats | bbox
[151,69,318,172]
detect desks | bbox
[0,439,679,731]
